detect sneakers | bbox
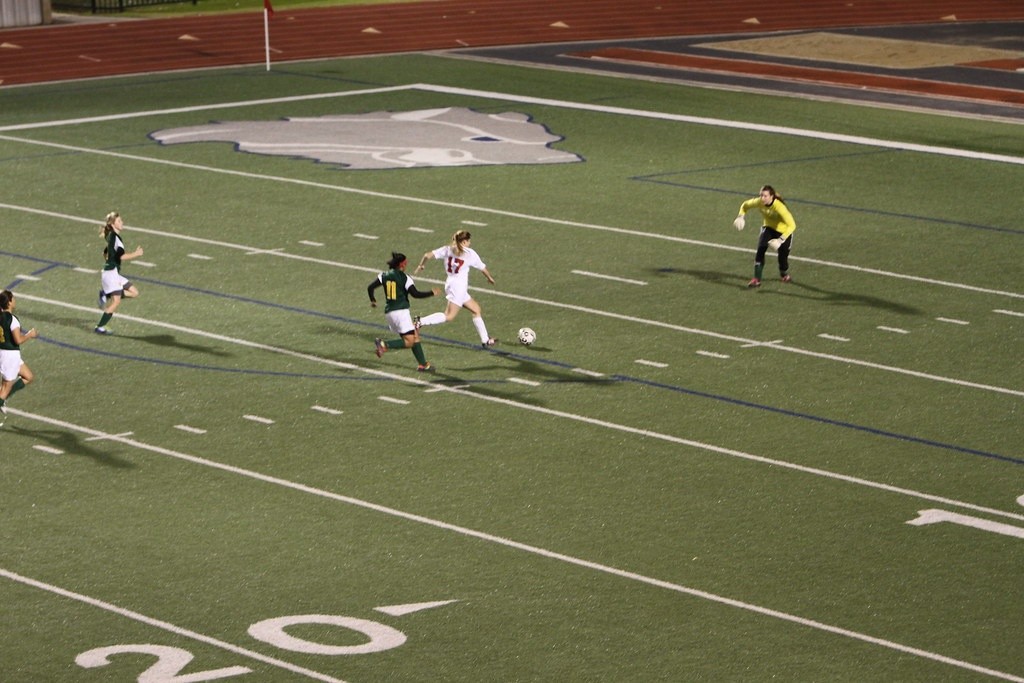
[413,316,422,334]
[482,337,499,348]
[416,362,432,372]
[99,290,106,310]
[375,338,387,358]
[94,325,114,335]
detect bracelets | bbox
[779,236,784,241]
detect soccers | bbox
[518,327,536,345]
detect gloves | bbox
[768,237,786,251]
[733,215,745,231]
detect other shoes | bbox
[782,275,791,282]
[1,402,7,413]
[748,279,761,287]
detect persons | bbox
[0,290,39,428]
[733,185,797,289]
[414,229,499,349]
[95,211,143,334]
[368,251,442,372]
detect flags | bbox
[264,0,273,15]
